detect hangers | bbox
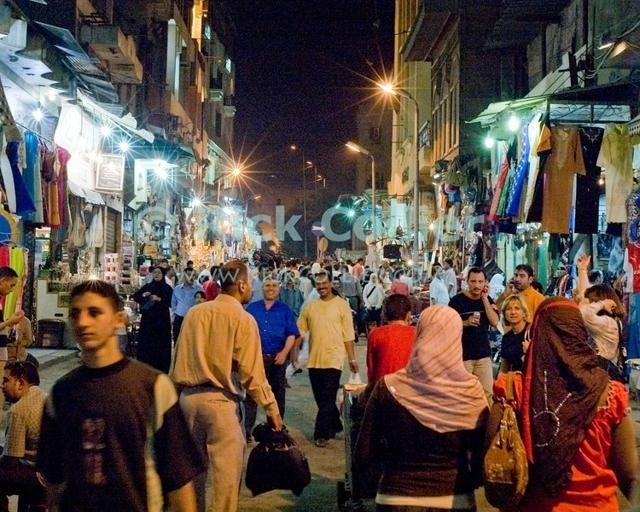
[0,199,20,224]
[0,231,29,253]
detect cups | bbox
[474,311,480,319]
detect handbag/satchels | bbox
[596,355,628,387]
[480,399,531,512]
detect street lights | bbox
[345,140,378,241]
[319,174,327,188]
[291,145,308,262]
[385,83,422,284]
[243,192,263,237]
[306,158,318,195]
[213,169,241,262]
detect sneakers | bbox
[313,423,344,448]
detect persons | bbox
[0,360,49,511]
[133,258,638,512]
[29,279,207,512]
[0,267,25,410]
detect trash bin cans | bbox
[37,319,65,349]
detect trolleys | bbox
[334,383,371,510]
